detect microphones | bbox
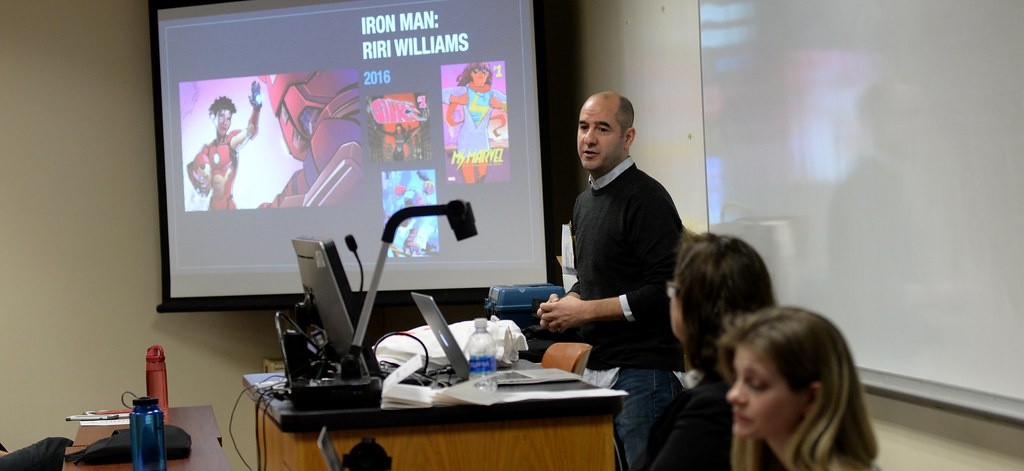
[344,235,365,295]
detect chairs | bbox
[542,342,593,376]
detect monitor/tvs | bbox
[279,235,383,385]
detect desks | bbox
[63,434,234,471]
[73,405,223,448]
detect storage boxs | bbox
[482,284,565,329]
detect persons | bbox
[0,435,75,471]
[627,232,777,471]
[712,305,882,471]
[536,89,687,471]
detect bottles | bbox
[145,344,169,425]
[121,391,168,471]
[468,318,498,393]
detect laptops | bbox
[412,292,582,387]
[317,426,341,471]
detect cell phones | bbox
[66,415,119,421]
